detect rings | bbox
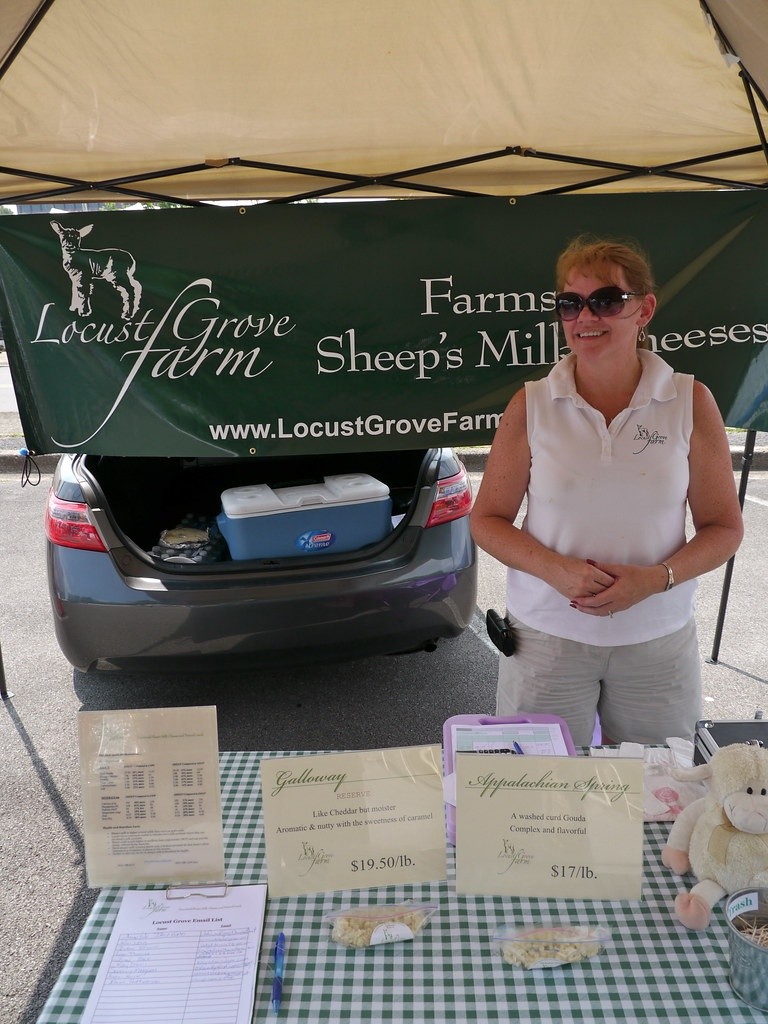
[593,594,596,597]
[609,612,613,619]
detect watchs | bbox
[658,562,675,593]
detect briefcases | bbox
[692,719,768,767]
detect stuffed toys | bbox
[662,743,768,931]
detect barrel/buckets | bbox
[724,886,768,1012]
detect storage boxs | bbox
[216,471,390,561]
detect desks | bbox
[37,745,768,1024]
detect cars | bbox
[43,445,481,675]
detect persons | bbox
[468,241,745,747]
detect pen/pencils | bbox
[512,739,524,754]
[271,931,288,1013]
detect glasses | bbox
[556,287,644,322]
